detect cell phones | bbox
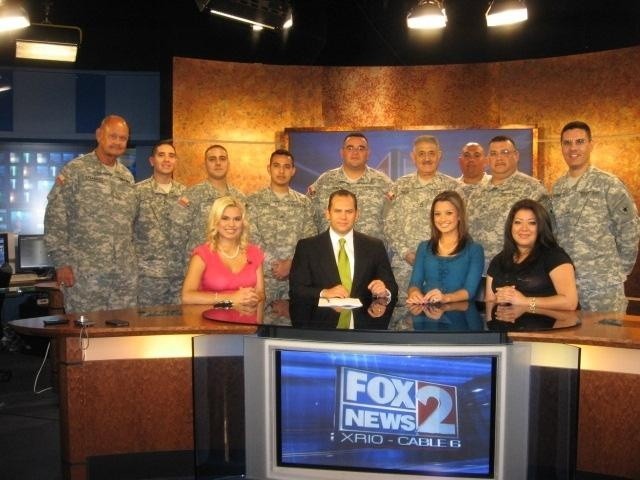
[44,319,69,325]
[106,319,130,327]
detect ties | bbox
[336,239,353,294]
[336,309,351,329]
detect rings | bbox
[378,309,381,314]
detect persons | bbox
[43,113,136,310]
[407,302,484,331]
[405,190,484,302]
[304,132,390,238]
[290,298,393,331]
[184,196,269,305]
[181,304,259,325]
[242,151,318,295]
[487,307,576,328]
[168,144,245,271]
[484,198,581,307]
[264,301,293,327]
[289,191,398,298]
[133,142,188,306]
[385,135,459,298]
[550,121,638,298]
[467,135,556,277]
[456,142,494,185]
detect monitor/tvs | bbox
[18,235,54,277]
[242,336,531,480]
[0,233,8,269]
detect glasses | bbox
[488,150,516,157]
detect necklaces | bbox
[438,239,457,252]
[213,242,243,264]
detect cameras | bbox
[74,316,95,327]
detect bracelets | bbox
[213,289,219,303]
[528,296,536,306]
[530,307,538,315]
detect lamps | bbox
[405,0,448,29]
[0,0,31,33]
[485,0,528,27]
[194,0,294,34]
[13,0,83,63]
